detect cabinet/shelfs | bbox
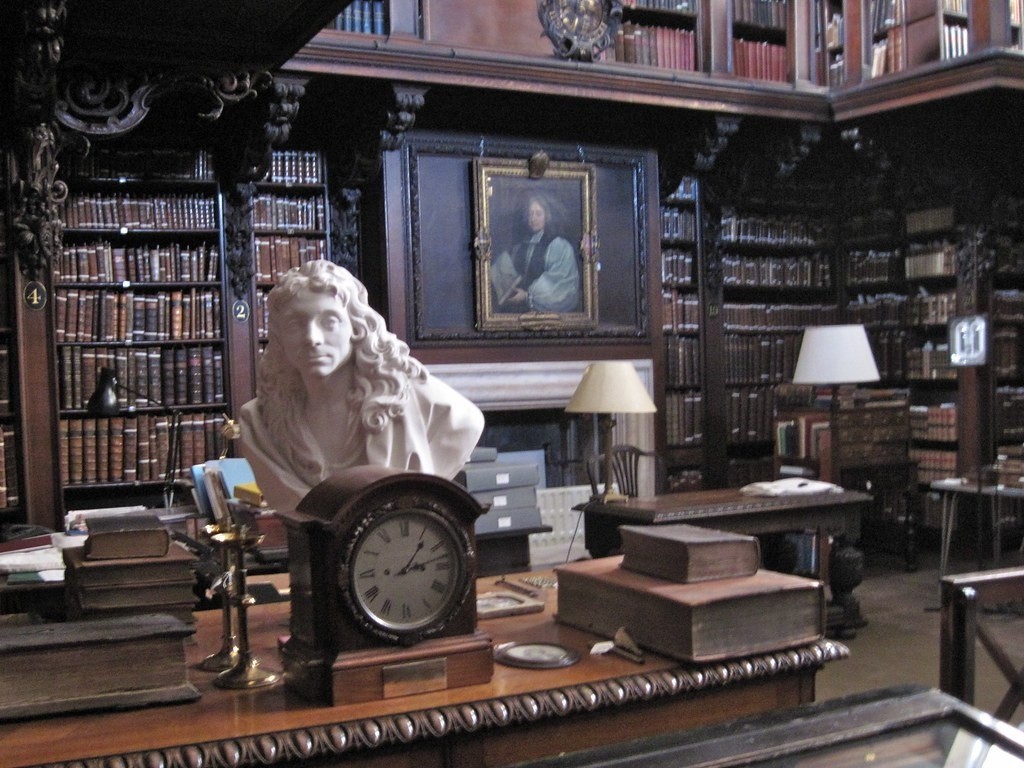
[719,205,841,449]
[840,199,958,552]
[1,134,332,533]
[616,0,1024,86]
[660,170,701,470]
[990,225,1024,449]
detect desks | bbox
[571,486,875,641]
[931,479,1024,595]
[0,524,555,613]
[1,571,852,768]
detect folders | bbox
[191,457,257,515]
[462,462,540,533]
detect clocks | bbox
[272,466,493,706]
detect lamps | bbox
[88,366,182,509]
[792,323,881,485]
[565,361,658,505]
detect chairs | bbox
[584,446,657,500]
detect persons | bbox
[237,257,487,517]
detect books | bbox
[0,0,1024,721]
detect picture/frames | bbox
[472,156,599,334]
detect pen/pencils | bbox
[589,641,646,663]
[502,580,537,598]
[798,482,808,488]
